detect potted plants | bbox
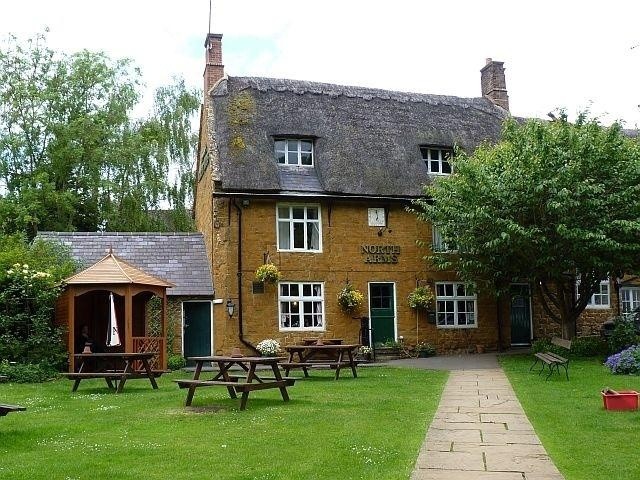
[415,341,432,358]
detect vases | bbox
[476,344,487,353]
[364,354,369,359]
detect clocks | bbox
[367,208,386,227]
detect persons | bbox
[74,326,103,372]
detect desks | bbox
[0,404,27,416]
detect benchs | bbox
[530,337,572,381]
[60,343,359,411]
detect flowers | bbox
[360,346,372,360]
[255,339,281,356]
[409,287,434,310]
[255,264,282,284]
[336,285,365,312]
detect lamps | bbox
[226,300,235,318]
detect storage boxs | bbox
[601,391,639,410]
